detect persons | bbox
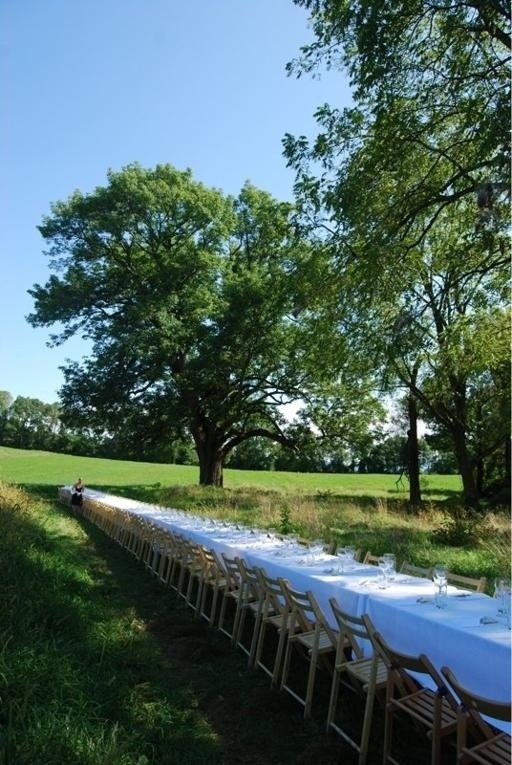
[70,477,84,517]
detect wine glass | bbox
[193,511,325,567]
[336,544,358,575]
[375,552,399,590]
[433,567,449,609]
[491,576,511,630]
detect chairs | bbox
[279,578,355,721]
[56,481,315,692]
[370,629,459,765]
[440,659,512,765]
[327,598,401,759]
